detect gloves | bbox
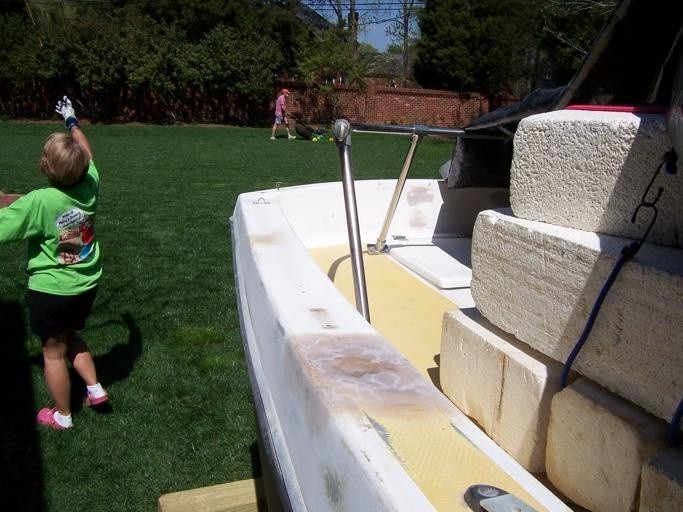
[55,96,79,130]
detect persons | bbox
[0,95,109,429]
[268,89,297,140]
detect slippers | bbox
[85,392,109,408]
[37,407,70,430]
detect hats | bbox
[282,89,290,94]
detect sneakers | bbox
[288,135,297,139]
[271,136,276,140]
